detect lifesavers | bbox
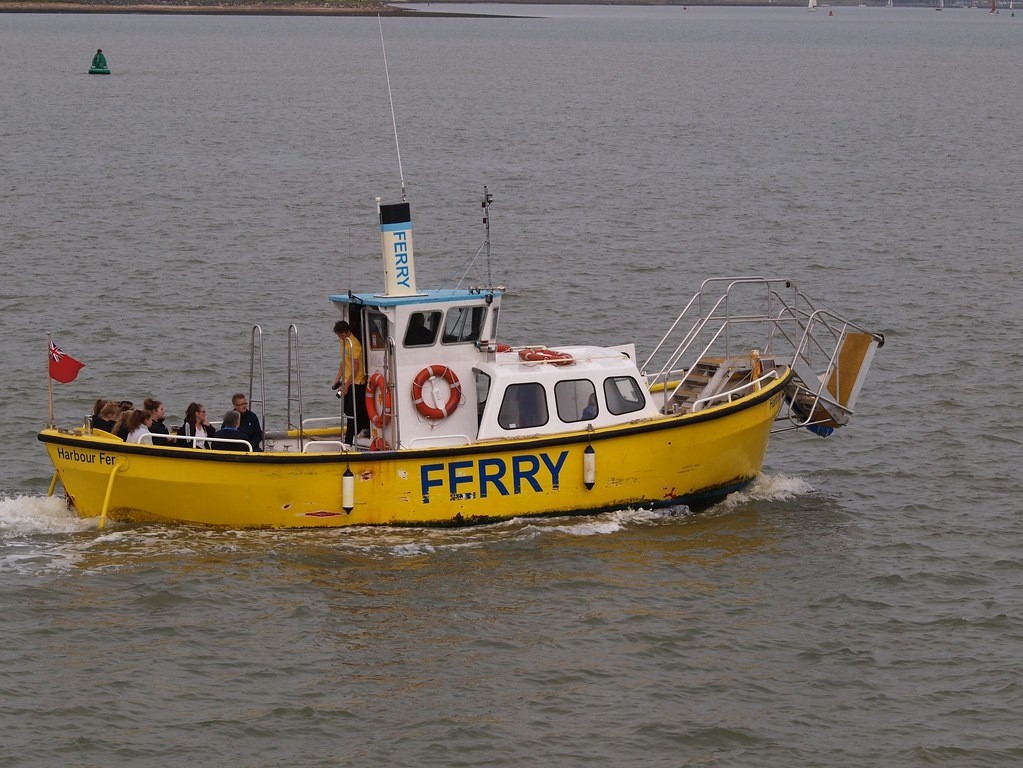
[411,364,463,421]
[517,348,573,366]
[365,372,394,429]
[370,436,392,452]
[496,343,514,353]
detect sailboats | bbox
[808,0,817,11]
[989,0,999,13]
[936,0,944,11]
[885,0,894,8]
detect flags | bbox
[48,336,86,384]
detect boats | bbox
[36,16,881,532]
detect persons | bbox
[144,398,169,446]
[126,410,153,445]
[221,393,262,447]
[334,320,370,451]
[176,403,216,450]
[405,313,434,345]
[92,398,135,442]
[581,393,597,420]
[211,410,263,452]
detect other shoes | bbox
[355,428,370,438]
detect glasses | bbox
[236,402,248,406]
